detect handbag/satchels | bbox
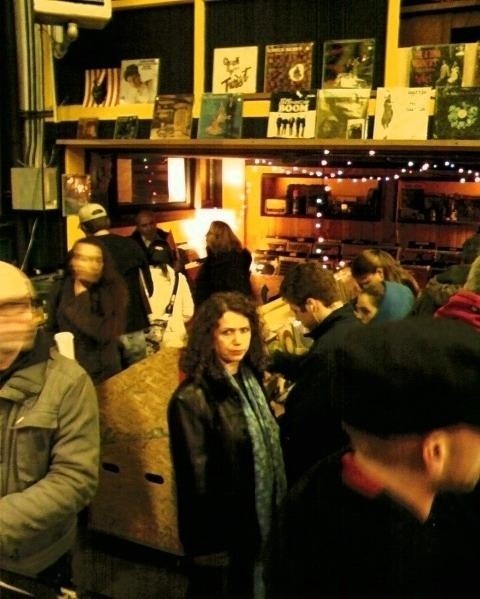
[145,318,168,356]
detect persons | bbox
[435,58,460,85]
[333,56,354,86]
[124,65,154,104]
[276,114,305,137]
[263,228,479,599]
[168,292,287,598]
[224,94,237,138]
[193,220,257,306]
[46,203,194,386]
[1,261,100,590]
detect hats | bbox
[342,315,479,437]
[78,203,106,227]
[149,240,170,255]
[0,260,36,302]
